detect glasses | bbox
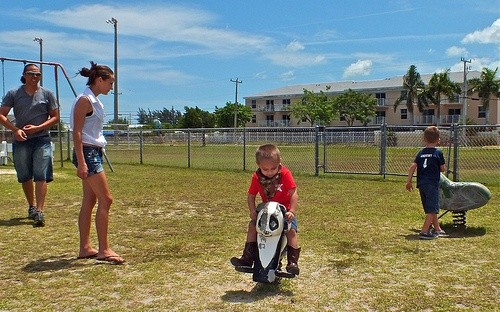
[24,72,41,77]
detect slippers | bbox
[98,255,125,264]
[77,251,98,259]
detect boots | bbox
[286,244,301,275]
[231,242,257,267]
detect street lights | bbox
[34,37,43,86]
[105,17,118,145]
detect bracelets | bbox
[290,211,295,215]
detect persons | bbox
[231,144,301,276]
[69,63,124,265]
[406,126,449,240]
[0,64,60,226]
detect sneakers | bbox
[28,207,37,219]
[34,211,44,225]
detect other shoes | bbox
[433,230,450,237]
[419,233,439,239]
